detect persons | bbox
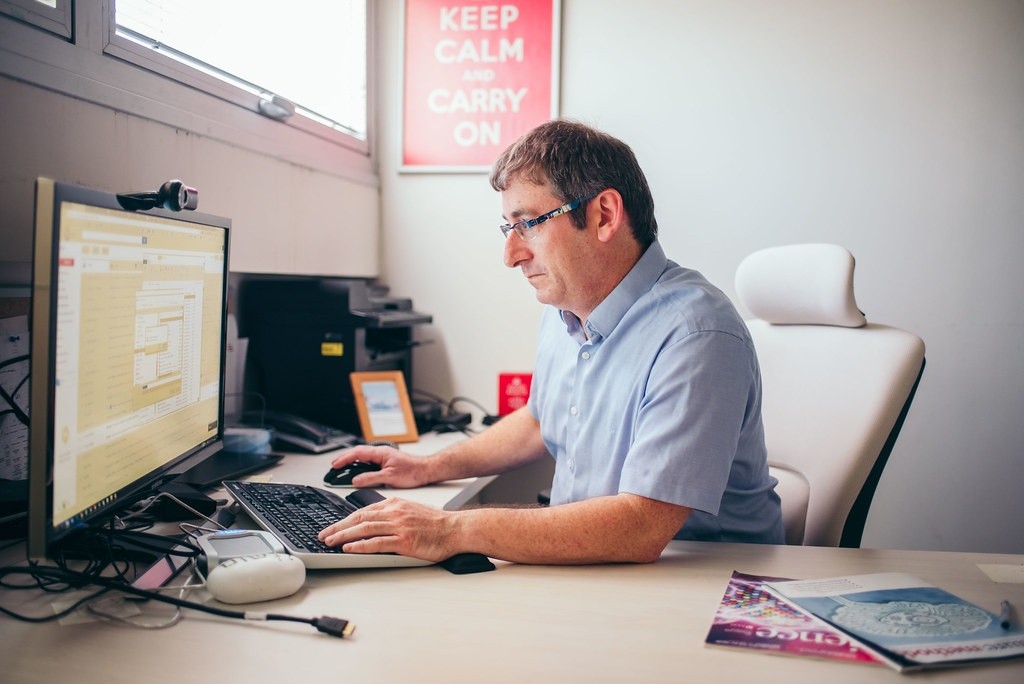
[317,120,787,566]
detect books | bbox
[704,570,880,664]
[761,571,1024,674]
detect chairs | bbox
[734,241,928,546]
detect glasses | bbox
[500,190,602,241]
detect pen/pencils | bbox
[999,600,1012,629]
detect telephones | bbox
[260,415,357,455]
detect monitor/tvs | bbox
[28,177,236,602]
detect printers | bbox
[237,276,434,437]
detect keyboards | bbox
[221,481,440,570]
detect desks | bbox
[0,410,1023,683]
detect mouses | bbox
[323,460,383,488]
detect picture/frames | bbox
[347,371,418,443]
